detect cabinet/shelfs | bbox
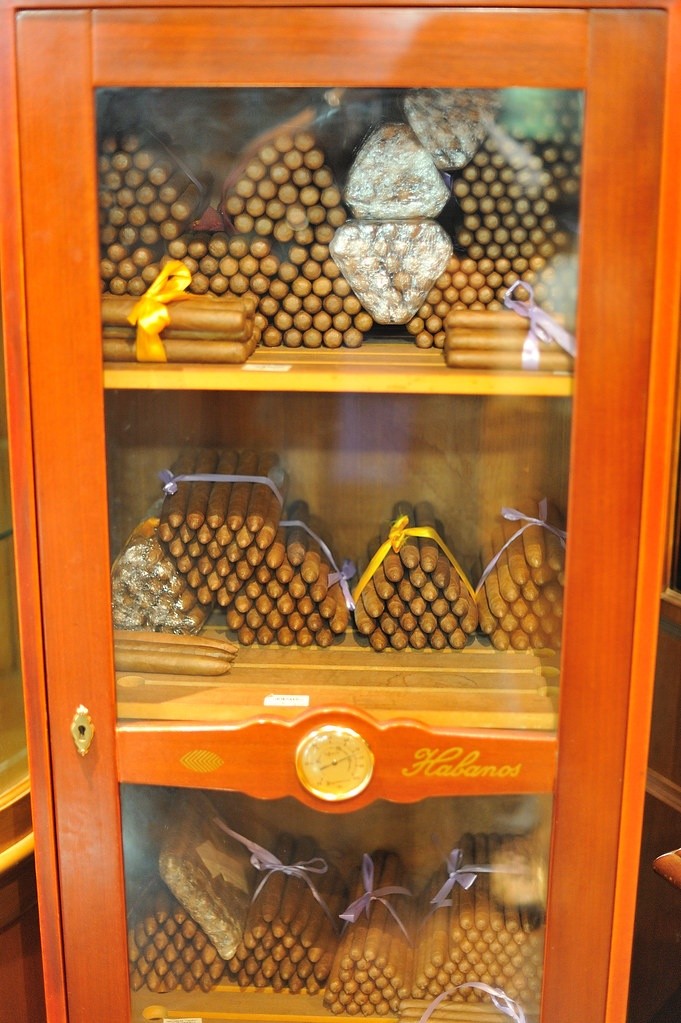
[1,0,679,1022]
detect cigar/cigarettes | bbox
[127,788,545,1023]
[94,86,583,371]
[110,437,565,676]
